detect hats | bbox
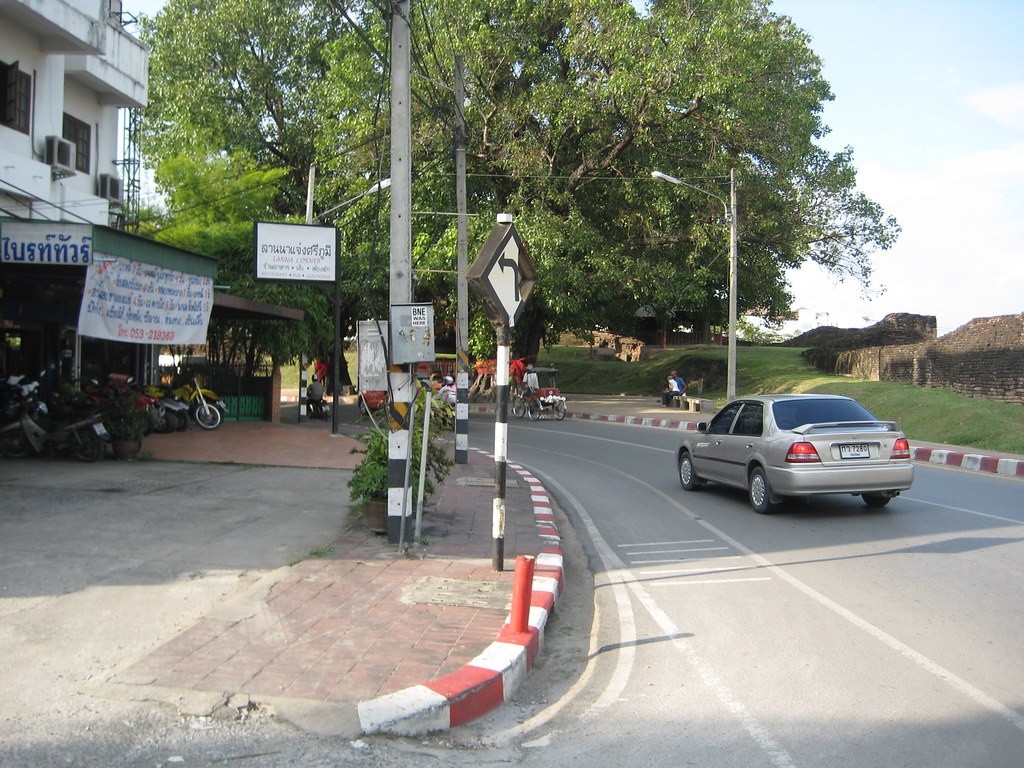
[525,364,534,371]
[312,374,318,382]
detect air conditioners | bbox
[44,136,76,174]
[99,174,124,205]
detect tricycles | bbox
[511,384,567,422]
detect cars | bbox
[677,393,917,516]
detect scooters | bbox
[305,392,330,423]
[0,347,225,463]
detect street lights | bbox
[650,165,741,403]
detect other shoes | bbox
[661,404,667,407]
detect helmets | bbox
[443,375,453,385]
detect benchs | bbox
[669,396,714,414]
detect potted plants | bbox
[345,460,434,535]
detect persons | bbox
[520,363,539,399]
[433,376,457,425]
[307,374,324,419]
[661,370,687,407]
